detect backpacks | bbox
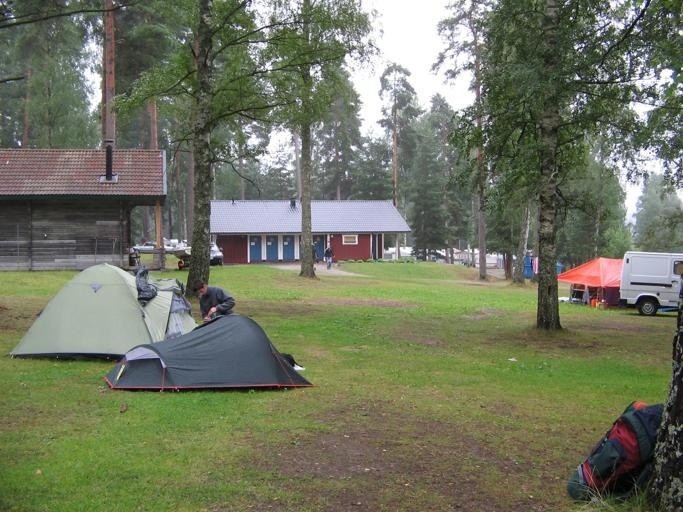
[567,401,664,501]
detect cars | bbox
[208,243,224,265]
[133,239,173,254]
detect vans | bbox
[618,251,683,316]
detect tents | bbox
[8,262,198,360]
[105,315,312,391]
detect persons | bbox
[191,278,236,323]
[322,245,335,269]
